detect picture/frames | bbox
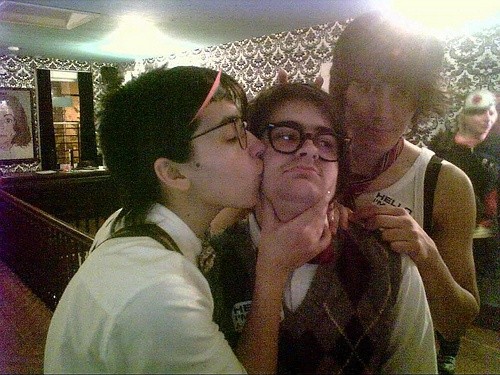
[0,86,37,163]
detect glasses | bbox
[256,123,352,163]
[189,117,248,151]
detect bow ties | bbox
[308,242,335,265]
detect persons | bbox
[43,8,500,375]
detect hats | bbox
[463,90,494,110]
